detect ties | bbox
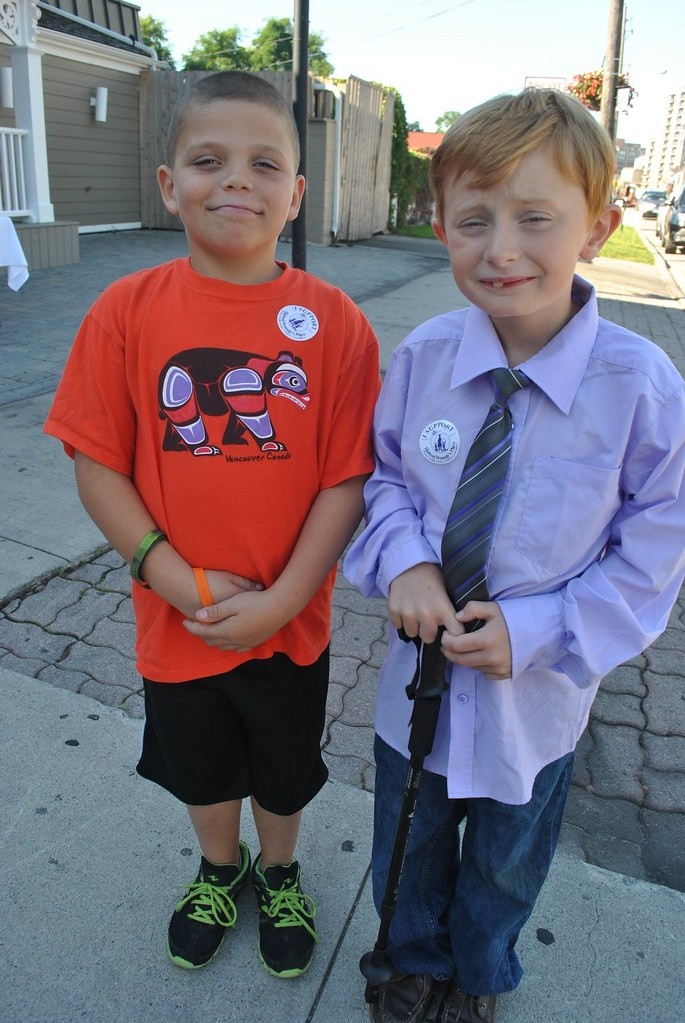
[435,366,533,636]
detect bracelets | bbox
[129,528,168,591]
[192,565,214,609]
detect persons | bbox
[336,83,683,1022]
[39,68,382,980]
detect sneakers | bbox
[248,847,316,982]
[365,966,436,1022]
[163,834,254,972]
[436,978,495,1023]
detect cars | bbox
[656,187,685,240]
[641,191,667,219]
[617,181,661,212]
[661,187,685,255]
